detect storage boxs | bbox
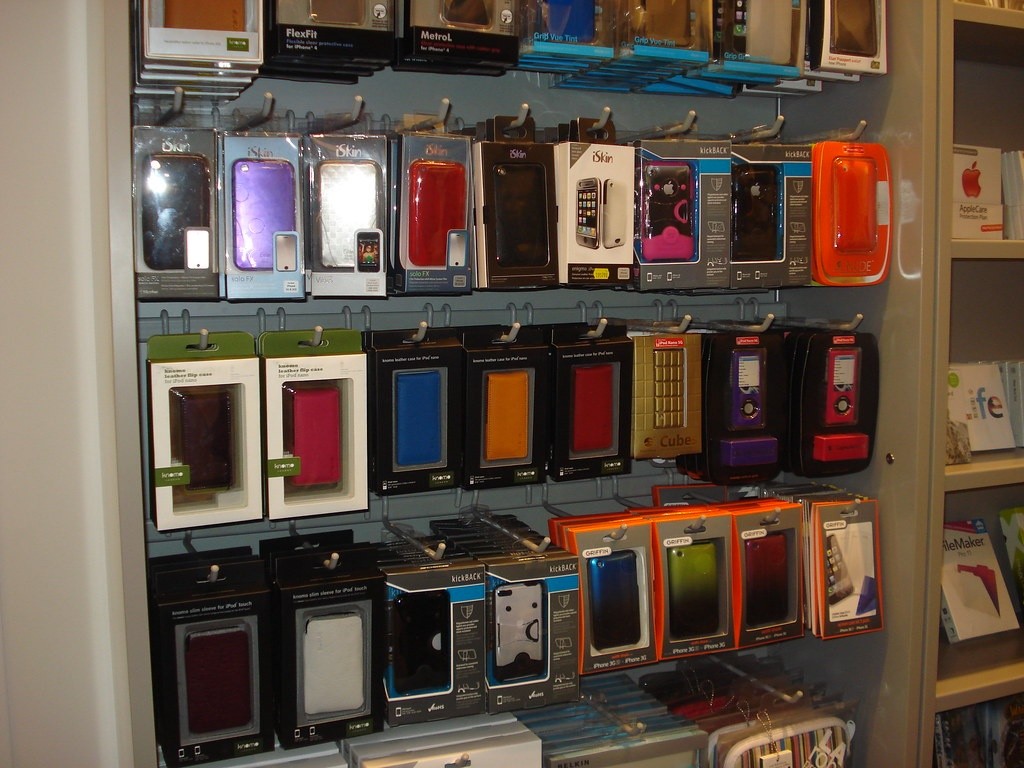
[130,2,894,768]
[938,141,1024,643]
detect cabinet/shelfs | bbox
[0,1,922,768]
[915,0,1024,768]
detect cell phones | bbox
[276,234,298,272]
[357,232,380,272]
[826,533,856,608]
[187,230,210,269]
[575,176,603,250]
[448,231,468,269]
[604,178,626,248]
